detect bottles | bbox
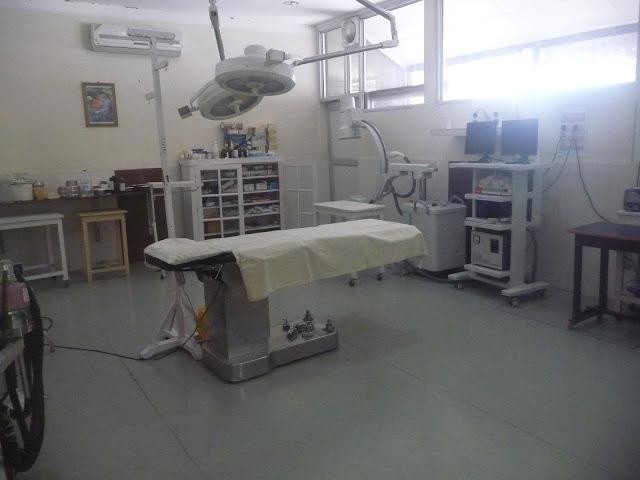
[242,144,248,157]
[235,144,241,157]
[222,144,231,157]
[34,178,43,198]
[213,141,219,159]
[101,180,107,190]
[80,169,93,197]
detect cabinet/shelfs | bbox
[448,161,551,307]
[178,153,284,242]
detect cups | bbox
[119,183,125,191]
[233,150,238,158]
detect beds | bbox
[145,217,428,384]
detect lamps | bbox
[337,94,389,204]
[215,0,399,97]
[177,0,263,120]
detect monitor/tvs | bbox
[501,119,539,164]
[464,120,496,163]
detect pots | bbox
[8,175,34,203]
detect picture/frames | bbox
[81,82,118,127]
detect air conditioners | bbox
[89,23,182,57]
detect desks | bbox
[568,222,639,332]
[1,193,120,290]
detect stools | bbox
[78,207,130,282]
[314,200,385,285]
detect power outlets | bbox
[559,112,584,150]
